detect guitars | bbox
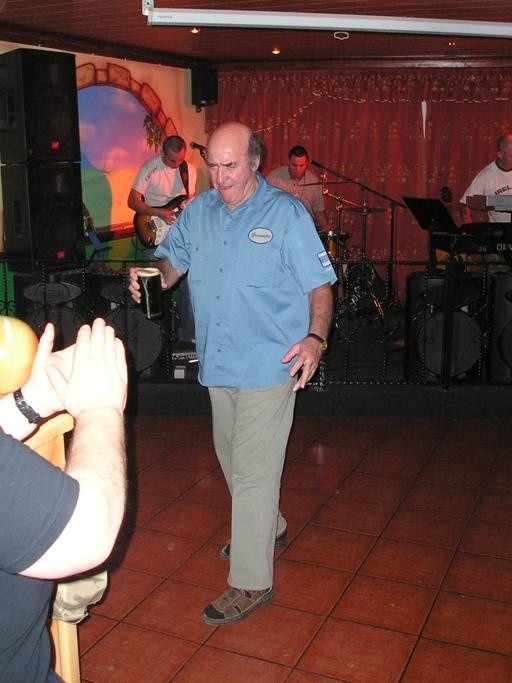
[134,193,190,249]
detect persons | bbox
[0,311,131,683]
[125,118,345,630]
[126,133,198,347]
[263,145,339,240]
[454,131,512,227]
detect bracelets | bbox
[11,386,46,424]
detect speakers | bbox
[0,165,88,273]
[191,67,219,106]
[407,270,511,385]
[0,47,82,164]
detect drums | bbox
[98,282,130,303]
[318,228,349,260]
[344,257,388,299]
[96,306,164,373]
[26,303,86,352]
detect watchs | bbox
[307,333,328,352]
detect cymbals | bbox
[297,178,351,186]
[342,204,387,214]
[23,280,81,304]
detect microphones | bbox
[189,139,207,154]
[309,159,325,169]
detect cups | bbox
[136,267,164,317]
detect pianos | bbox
[429,219,512,255]
[463,192,512,212]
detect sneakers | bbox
[201,582,274,625]
[219,525,288,560]
